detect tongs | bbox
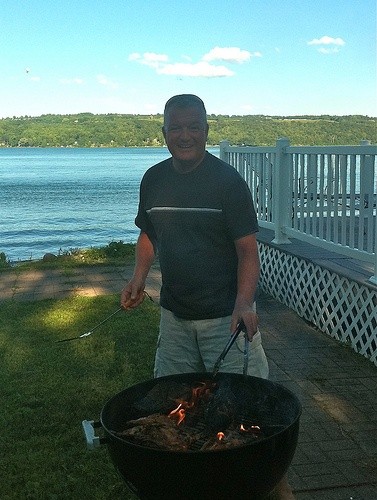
[210,320,249,385]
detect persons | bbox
[120,95,296,500]
